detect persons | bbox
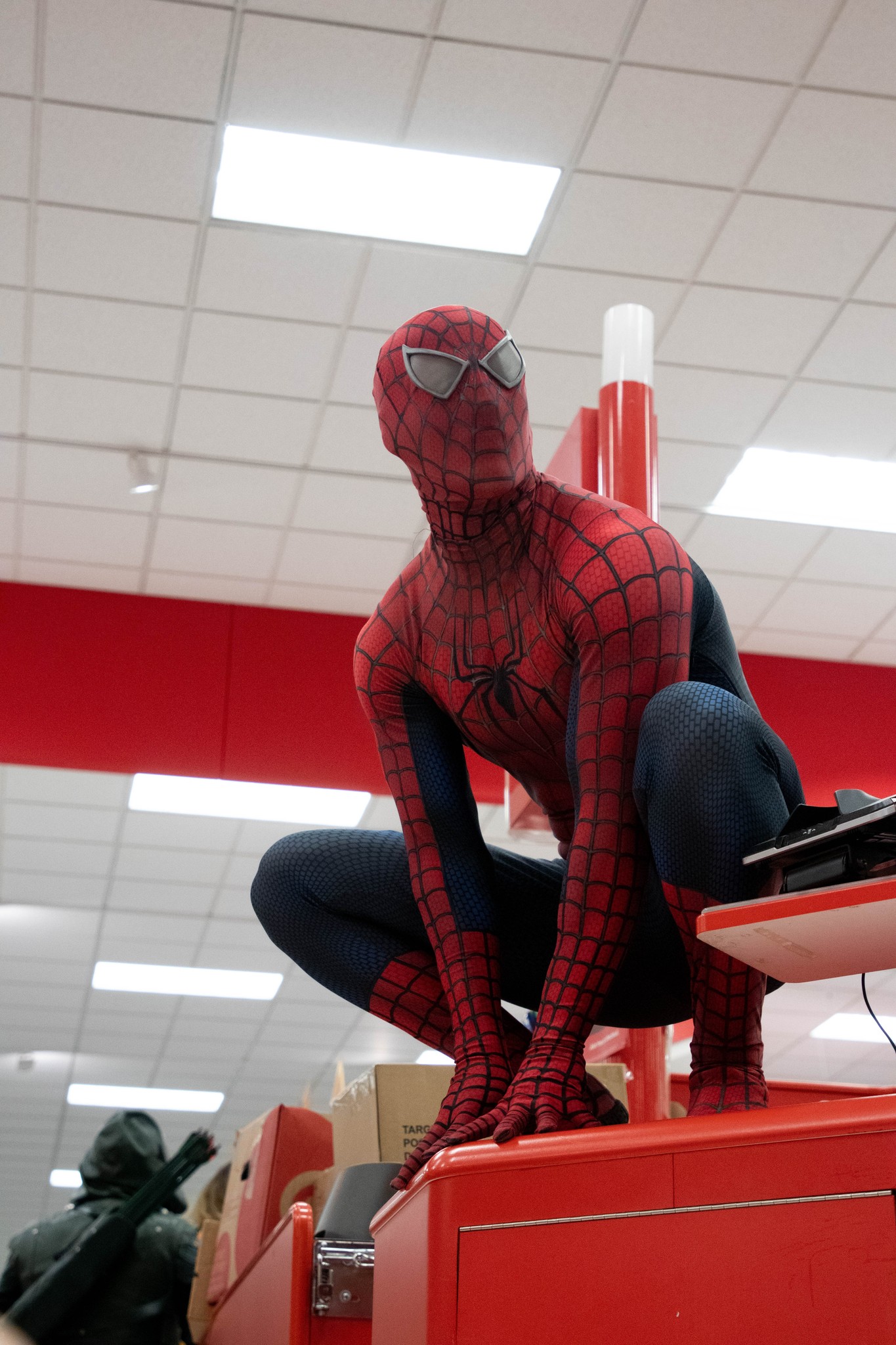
[250,302,805,1192]
[0,1109,199,1345]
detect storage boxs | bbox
[204,1102,337,1309]
[326,1059,631,1184]
[184,1217,221,1345]
